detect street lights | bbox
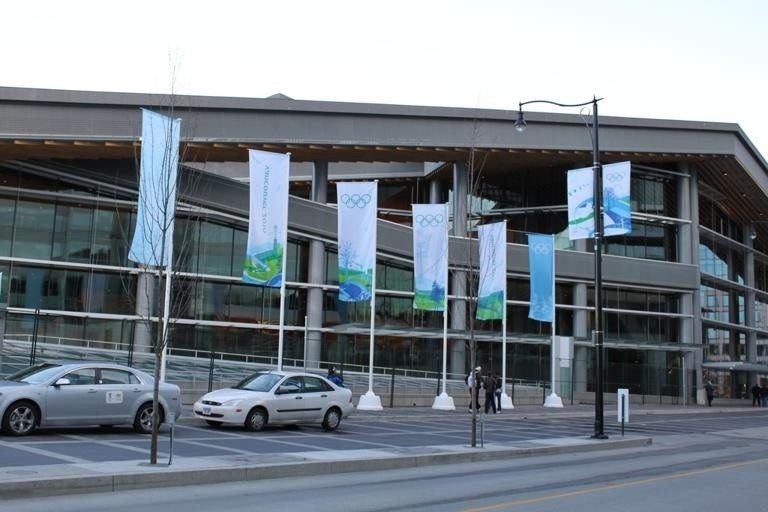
[514,95,612,440]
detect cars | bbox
[0,360,182,436]
[193,371,355,431]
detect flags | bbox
[602,160,633,237]
[127,108,180,267]
[336,180,377,304]
[566,164,596,241]
[240,148,291,290]
[526,234,554,322]
[410,201,449,312]
[475,221,506,321]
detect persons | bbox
[760,385,767,407]
[467,365,503,414]
[752,383,761,408]
[706,379,714,406]
[327,369,345,387]
[329,366,339,374]
